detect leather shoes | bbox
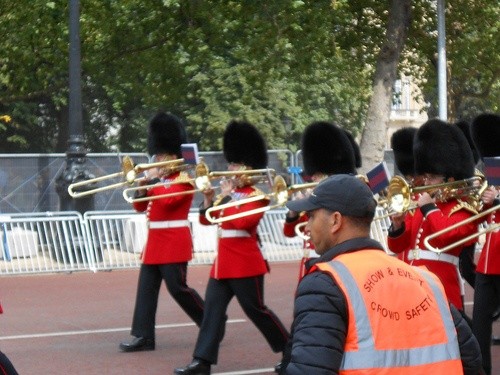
[120,336,155,351]
[219,315,227,341]
[173,359,211,375]
[274,337,293,373]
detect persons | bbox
[286,173,484,375]
[119,111,499,374]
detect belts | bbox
[146,220,190,229]
[301,249,321,258]
[407,248,459,266]
[487,226,500,232]
[216,229,251,238]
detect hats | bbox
[302,122,355,174]
[342,129,362,168]
[470,113,500,157]
[286,174,376,217]
[391,127,420,176]
[223,120,268,169]
[455,121,479,165]
[147,112,187,158]
[413,119,474,177]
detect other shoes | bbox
[492,336,500,345]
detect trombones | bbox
[204,174,370,223]
[121,159,277,204]
[295,199,419,241]
[423,204,500,254]
[372,175,483,222]
[67,154,205,200]
